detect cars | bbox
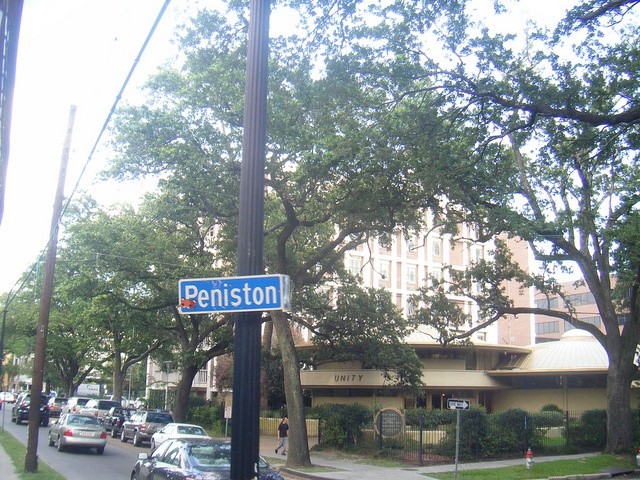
[47,413,108,454]
[47,396,69,416]
[128,437,284,480]
[150,423,211,450]
[0,391,14,403]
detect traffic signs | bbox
[447,398,470,410]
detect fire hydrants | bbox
[525,446,535,469]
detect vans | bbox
[80,399,122,422]
[61,396,91,414]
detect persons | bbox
[274,417,289,455]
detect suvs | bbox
[10,393,50,426]
[120,407,175,448]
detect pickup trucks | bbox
[101,406,137,438]
